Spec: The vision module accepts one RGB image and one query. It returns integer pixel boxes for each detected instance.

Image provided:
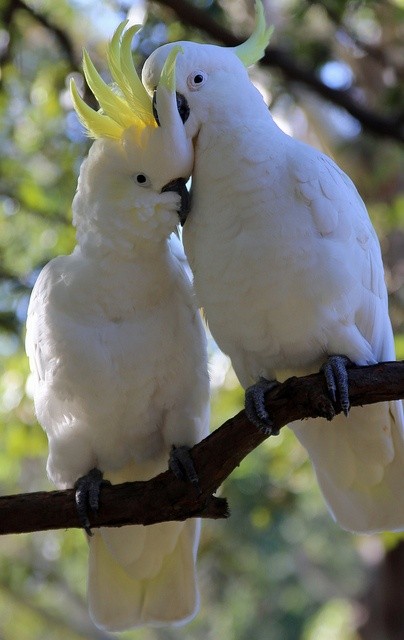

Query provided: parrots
[21,16,214,633]
[140,0,403,542]
